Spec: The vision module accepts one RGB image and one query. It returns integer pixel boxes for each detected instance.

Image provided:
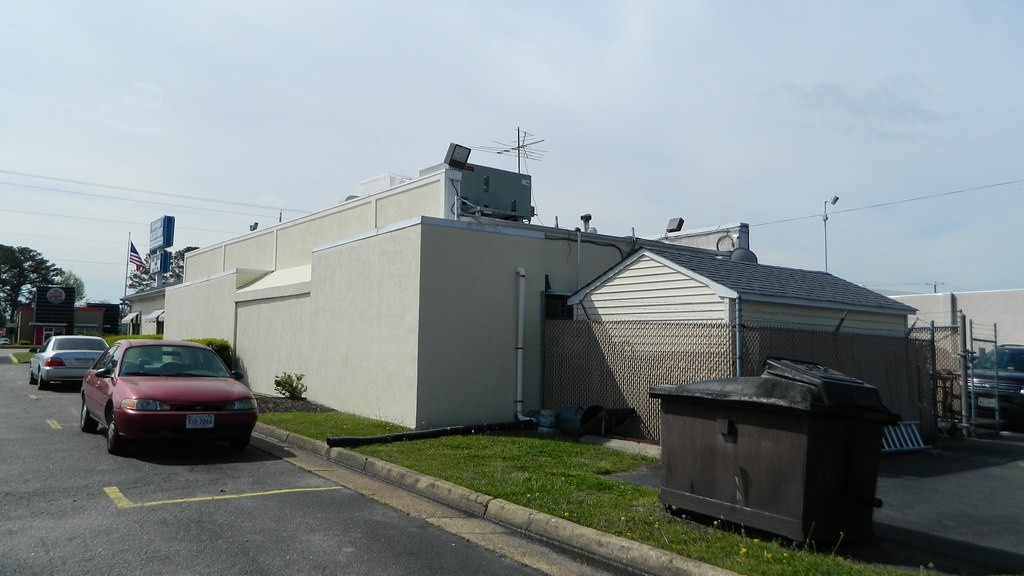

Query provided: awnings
[144,309,165,322]
[121,312,140,324]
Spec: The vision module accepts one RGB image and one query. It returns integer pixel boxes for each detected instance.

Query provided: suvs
[965,343,1024,424]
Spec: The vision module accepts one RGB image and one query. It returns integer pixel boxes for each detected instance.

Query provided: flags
[129,241,146,273]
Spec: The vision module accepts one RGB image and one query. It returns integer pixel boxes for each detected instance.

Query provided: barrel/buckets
[537,409,557,433]
[559,404,608,436]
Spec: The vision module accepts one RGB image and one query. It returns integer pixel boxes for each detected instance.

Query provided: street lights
[822,195,841,271]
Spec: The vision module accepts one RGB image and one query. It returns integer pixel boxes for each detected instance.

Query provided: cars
[78,339,259,457]
[0,338,11,345]
[28,335,112,390]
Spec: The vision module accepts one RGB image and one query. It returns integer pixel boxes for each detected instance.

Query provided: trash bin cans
[649,356,883,546]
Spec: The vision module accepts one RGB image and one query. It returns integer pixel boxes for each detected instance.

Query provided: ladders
[880,421,932,453]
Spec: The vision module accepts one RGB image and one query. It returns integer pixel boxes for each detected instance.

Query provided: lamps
[665,217,684,234]
[443,142,471,169]
